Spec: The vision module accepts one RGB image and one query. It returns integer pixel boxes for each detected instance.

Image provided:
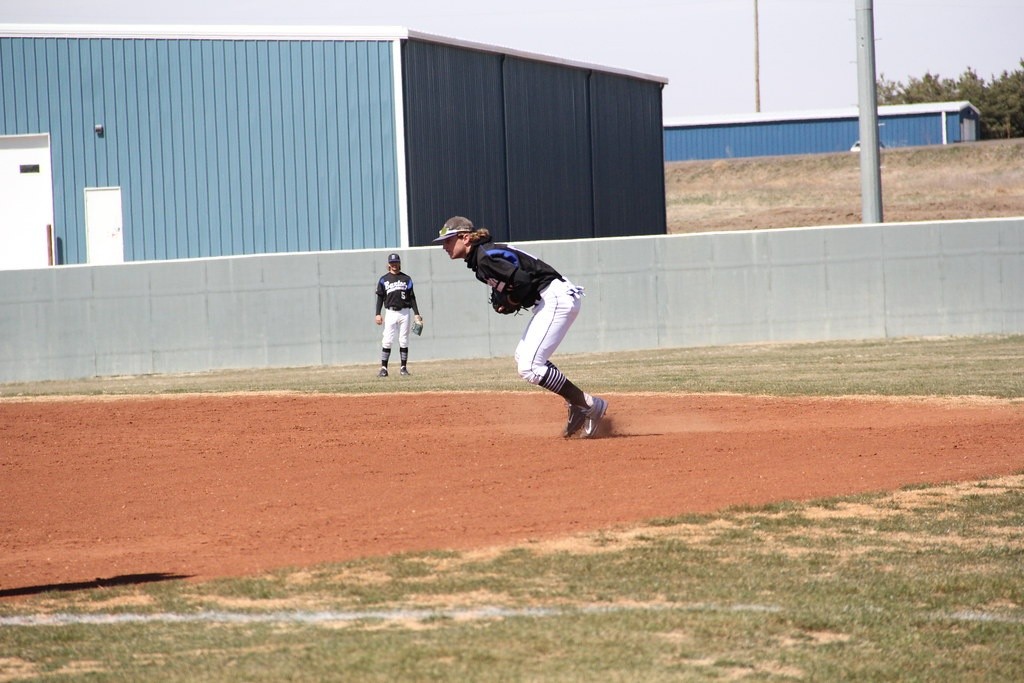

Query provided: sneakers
[562,400,586,437]
[577,396,608,438]
[377,368,388,377]
[400,368,410,375]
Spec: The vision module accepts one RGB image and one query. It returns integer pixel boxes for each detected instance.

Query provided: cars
[850,139,884,150]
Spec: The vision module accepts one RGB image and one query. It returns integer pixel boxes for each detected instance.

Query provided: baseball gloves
[411,315,424,337]
[489,286,520,316]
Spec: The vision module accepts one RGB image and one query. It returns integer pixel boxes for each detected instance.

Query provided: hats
[388,254,400,262]
[432,216,474,245]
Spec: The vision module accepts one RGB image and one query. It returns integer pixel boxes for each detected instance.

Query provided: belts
[388,306,402,311]
[534,277,566,306]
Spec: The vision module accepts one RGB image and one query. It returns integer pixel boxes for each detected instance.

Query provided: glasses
[440,229,470,236]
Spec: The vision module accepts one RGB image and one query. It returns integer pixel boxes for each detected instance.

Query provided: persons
[432,215,608,439]
[376,253,422,377]
